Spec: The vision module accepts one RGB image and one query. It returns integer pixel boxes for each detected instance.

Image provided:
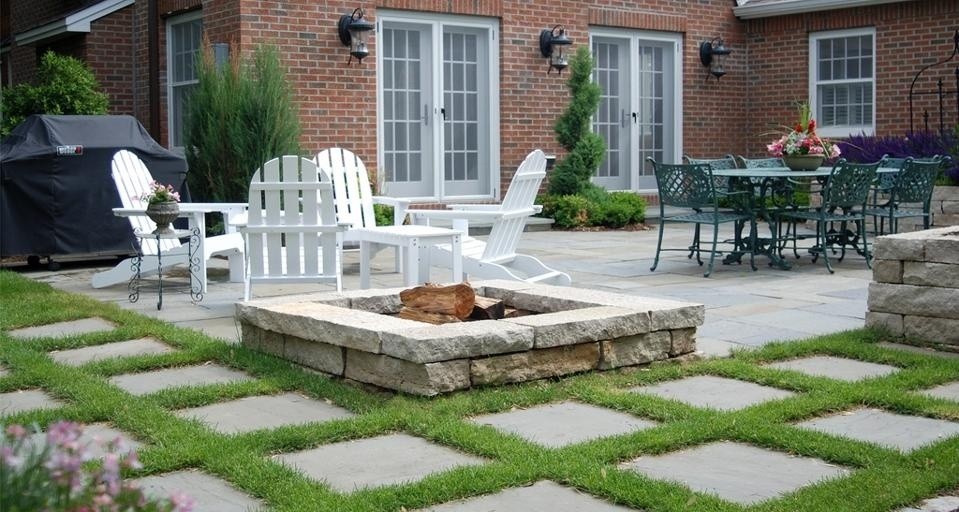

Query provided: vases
[781,155,825,171]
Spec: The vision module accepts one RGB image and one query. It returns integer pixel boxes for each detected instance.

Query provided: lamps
[699,36,733,83]
[537,23,574,78]
[336,5,376,68]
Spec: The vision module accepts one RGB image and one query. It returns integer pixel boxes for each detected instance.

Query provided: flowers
[753,89,844,164]
